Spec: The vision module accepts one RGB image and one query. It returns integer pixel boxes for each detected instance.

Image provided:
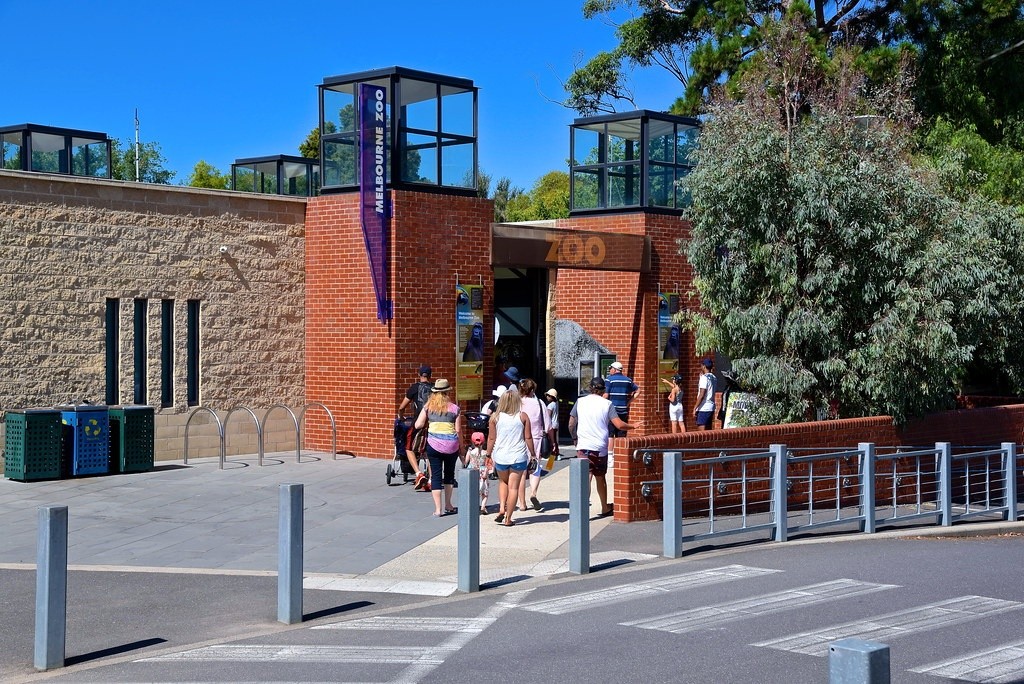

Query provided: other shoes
[433,507,458,518]
[607,455,614,462]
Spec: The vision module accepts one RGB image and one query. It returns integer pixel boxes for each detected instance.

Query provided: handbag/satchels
[541,436,550,458]
[411,428,428,452]
[528,456,537,474]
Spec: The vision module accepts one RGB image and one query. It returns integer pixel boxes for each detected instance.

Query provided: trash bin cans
[55,404,111,477]
[107,405,155,475]
[4,409,62,484]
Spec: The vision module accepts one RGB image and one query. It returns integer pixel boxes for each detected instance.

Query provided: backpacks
[418,381,433,409]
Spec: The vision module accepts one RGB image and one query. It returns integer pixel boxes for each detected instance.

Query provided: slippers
[602,503,614,516]
[494,497,541,526]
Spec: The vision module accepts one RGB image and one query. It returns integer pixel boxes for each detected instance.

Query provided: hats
[431,379,453,393]
[492,385,508,398]
[671,374,682,382]
[609,362,623,371]
[471,431,485,445]
[544,389,557,400]
[590,377,605,390]
[419,366,431,376]
[504,367,519,381]
[703,359,712,368]
[721,369,736,382]
[495,356,508,364]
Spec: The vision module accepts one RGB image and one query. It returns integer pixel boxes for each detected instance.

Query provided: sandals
[480,507,488,515]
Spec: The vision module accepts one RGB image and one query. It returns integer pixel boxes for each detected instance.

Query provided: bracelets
[460,445,465,449]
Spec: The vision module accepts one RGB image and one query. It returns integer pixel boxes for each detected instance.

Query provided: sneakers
[414,473,432,491]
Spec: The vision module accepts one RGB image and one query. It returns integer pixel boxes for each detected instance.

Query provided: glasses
[609,367,614,370]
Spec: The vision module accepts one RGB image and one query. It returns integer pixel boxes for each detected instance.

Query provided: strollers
[463,412,498,481]
[386,417,429,485]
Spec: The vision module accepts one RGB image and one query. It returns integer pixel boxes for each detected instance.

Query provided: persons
[414,379,466,517]
[464,363,559,527]
[661,374,686,434]
[716,369,743,429]
[602,362,641,468]
[693,358,718,431]
[398,366,434,490]
[567,377,644,517]
